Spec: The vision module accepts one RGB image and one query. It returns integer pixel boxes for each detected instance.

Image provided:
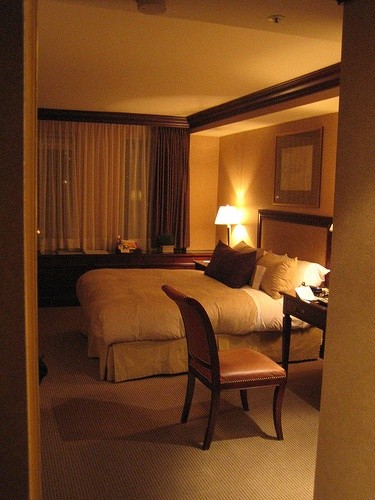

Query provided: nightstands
[278,284,330,374]
[192,258,212,269]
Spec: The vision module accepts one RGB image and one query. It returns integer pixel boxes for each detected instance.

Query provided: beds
[77,209,334,382]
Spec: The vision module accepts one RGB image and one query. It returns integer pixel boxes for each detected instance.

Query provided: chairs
[161,284,288,450]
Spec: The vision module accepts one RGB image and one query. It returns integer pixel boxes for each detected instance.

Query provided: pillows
[202,240,330,298]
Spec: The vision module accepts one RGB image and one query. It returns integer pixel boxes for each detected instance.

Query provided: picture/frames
[273,124,323,208]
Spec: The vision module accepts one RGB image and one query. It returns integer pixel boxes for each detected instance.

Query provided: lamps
[215,206,244,246]
